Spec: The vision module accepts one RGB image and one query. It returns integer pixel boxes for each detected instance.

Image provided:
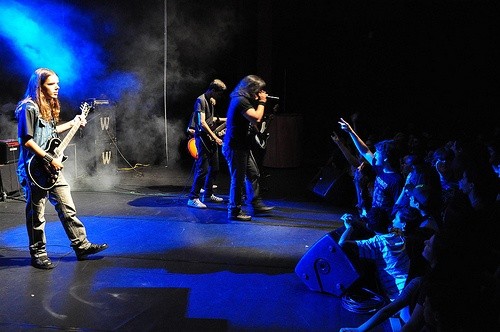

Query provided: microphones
[266,95,279,101]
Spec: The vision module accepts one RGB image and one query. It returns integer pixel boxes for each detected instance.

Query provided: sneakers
[207,194,223,202]
[187,198,207,208]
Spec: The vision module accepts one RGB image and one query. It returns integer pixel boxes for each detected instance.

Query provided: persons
[330,112,500,332]
[218,74,276,221]
[15,66,111,270]
[186,79,227,209]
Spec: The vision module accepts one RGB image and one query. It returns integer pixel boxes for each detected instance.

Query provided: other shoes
[251,206,274,214]
[32,256,55,268]
[227,213,251,220]
[73,243,108,261]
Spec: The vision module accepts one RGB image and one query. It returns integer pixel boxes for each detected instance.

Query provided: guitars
[188,128,226,159]
[255,103,279,149]
[28,102,90,190]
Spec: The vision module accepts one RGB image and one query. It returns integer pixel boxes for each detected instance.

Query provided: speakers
[83,104,117,175]
[295,220,378,296]
[0,162,21,195]
[312,172,357,209]
